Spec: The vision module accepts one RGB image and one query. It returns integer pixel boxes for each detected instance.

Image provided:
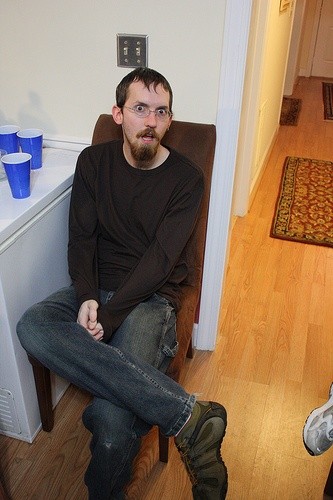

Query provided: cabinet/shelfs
[0,140,91,444]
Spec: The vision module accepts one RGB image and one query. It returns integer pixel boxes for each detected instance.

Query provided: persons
[18,66,227,500]
[302,380,333,500]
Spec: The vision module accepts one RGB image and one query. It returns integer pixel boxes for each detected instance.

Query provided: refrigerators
[0,134,92,446]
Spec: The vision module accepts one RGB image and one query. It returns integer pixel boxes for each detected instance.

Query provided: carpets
[280,96,302,126]
[322,81,333,120]
[270,156,333,248]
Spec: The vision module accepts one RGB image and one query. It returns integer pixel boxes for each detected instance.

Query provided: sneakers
[174,401,228,500]
[302,382,333,456]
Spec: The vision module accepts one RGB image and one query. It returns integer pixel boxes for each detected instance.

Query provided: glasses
[122,105,174,119]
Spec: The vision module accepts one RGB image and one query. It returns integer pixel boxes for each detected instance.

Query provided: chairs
[32,114,216,464]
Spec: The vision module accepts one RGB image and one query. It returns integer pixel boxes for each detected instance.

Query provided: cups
[0,125,21,154]
[1,152,33,199]
[0,149,8,182]
[16,129,43,170]
